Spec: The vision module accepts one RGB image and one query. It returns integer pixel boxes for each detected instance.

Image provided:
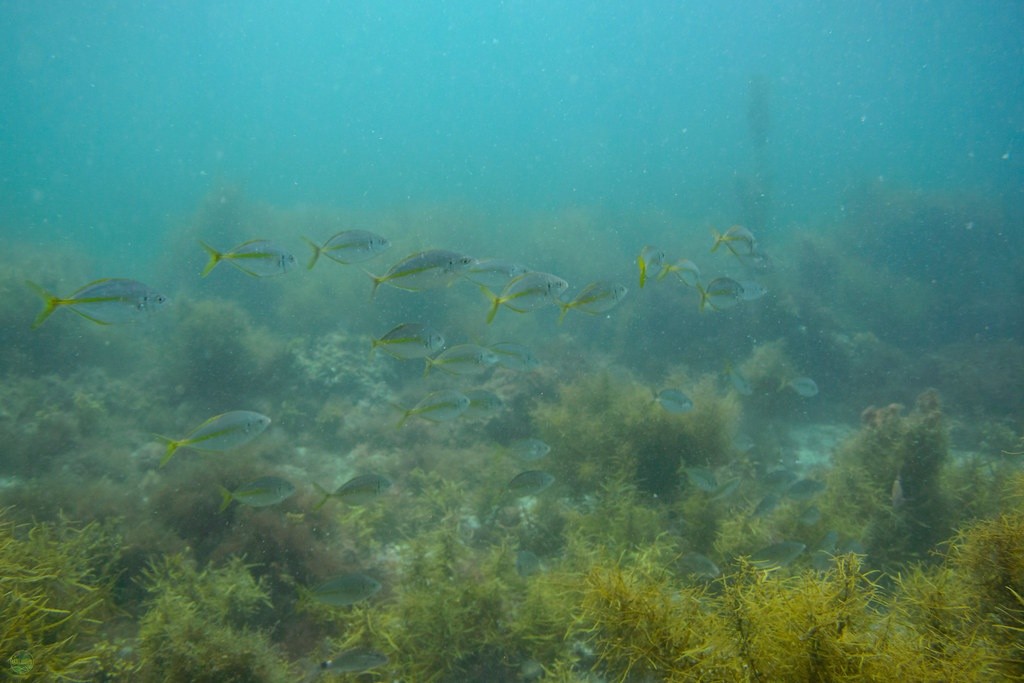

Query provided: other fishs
[297,223,828,683]
[312,475,392,515]
[392,389,471,432]
[198,240,300,282]
[369,322,445,360]
[304,229,392,272]
[153,410,271,468]
[214,475,298,516]
[24,278,166,330]
[365,248,479,297]
